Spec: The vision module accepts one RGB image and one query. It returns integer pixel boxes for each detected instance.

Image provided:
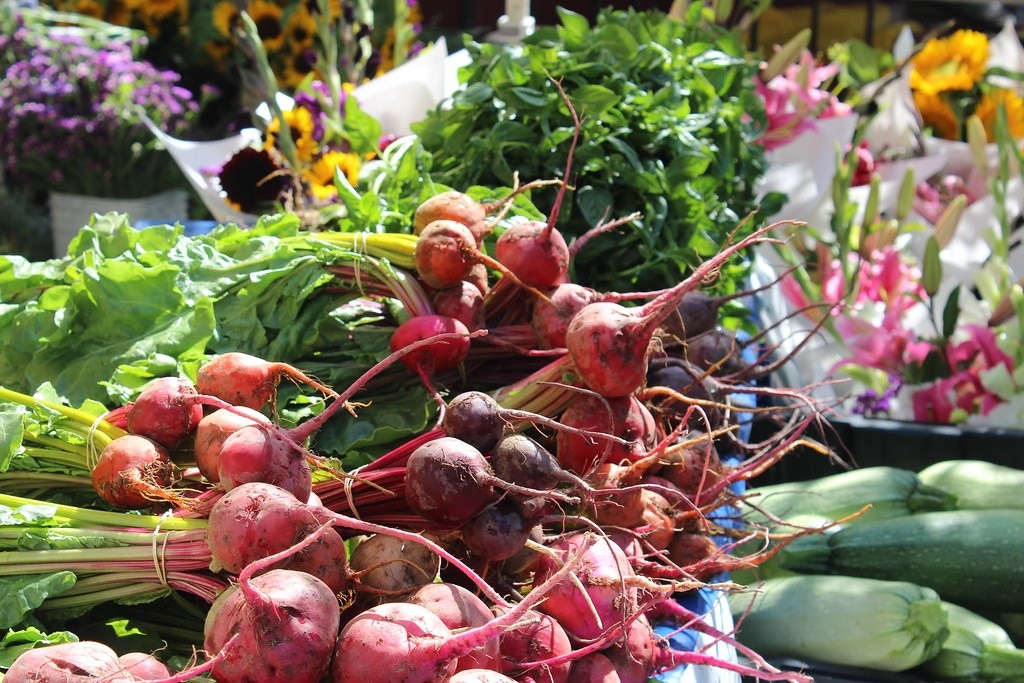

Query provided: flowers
[0,0,425,217]
[752,21,1023,426]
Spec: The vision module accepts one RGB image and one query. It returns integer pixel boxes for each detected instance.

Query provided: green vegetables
[394,1,792,305]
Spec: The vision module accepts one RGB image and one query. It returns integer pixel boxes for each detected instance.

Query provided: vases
[50,190,189,260]
